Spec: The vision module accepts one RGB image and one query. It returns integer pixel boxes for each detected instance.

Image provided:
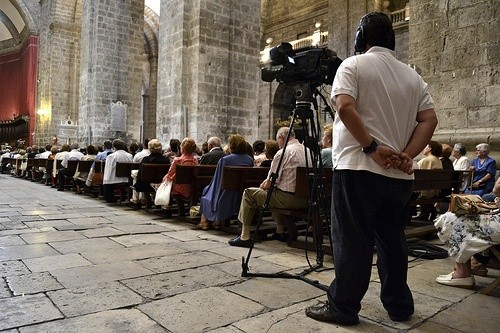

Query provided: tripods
[241,104,334,276]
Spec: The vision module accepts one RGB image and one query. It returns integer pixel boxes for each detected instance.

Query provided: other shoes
[196,223,208,230]
[229,236,254,247]
[271,233,288,242]
[435,271,476,289]
[470,264,488,277]
[50,185,56,188]
[130,198,140,203]
[413,213,429,220]
[210,223,220,230]
[75,191,82,194]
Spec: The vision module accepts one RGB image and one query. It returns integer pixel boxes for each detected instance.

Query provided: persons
[305,13,439,324]
[0,122,500,290]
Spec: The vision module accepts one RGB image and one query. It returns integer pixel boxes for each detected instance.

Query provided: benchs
[1,157,465,255]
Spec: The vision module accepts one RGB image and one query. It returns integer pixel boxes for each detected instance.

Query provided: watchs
[361,139,379,156]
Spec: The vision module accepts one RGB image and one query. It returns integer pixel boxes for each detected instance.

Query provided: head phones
[355,13,396,52]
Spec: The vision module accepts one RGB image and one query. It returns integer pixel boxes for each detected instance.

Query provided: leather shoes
[305,299,360,326]
[387,311,411,321]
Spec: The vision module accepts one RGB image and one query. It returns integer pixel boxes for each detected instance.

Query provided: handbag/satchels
[448,194,498,215]
[190,205,200,215]
[155,182,172,205]
